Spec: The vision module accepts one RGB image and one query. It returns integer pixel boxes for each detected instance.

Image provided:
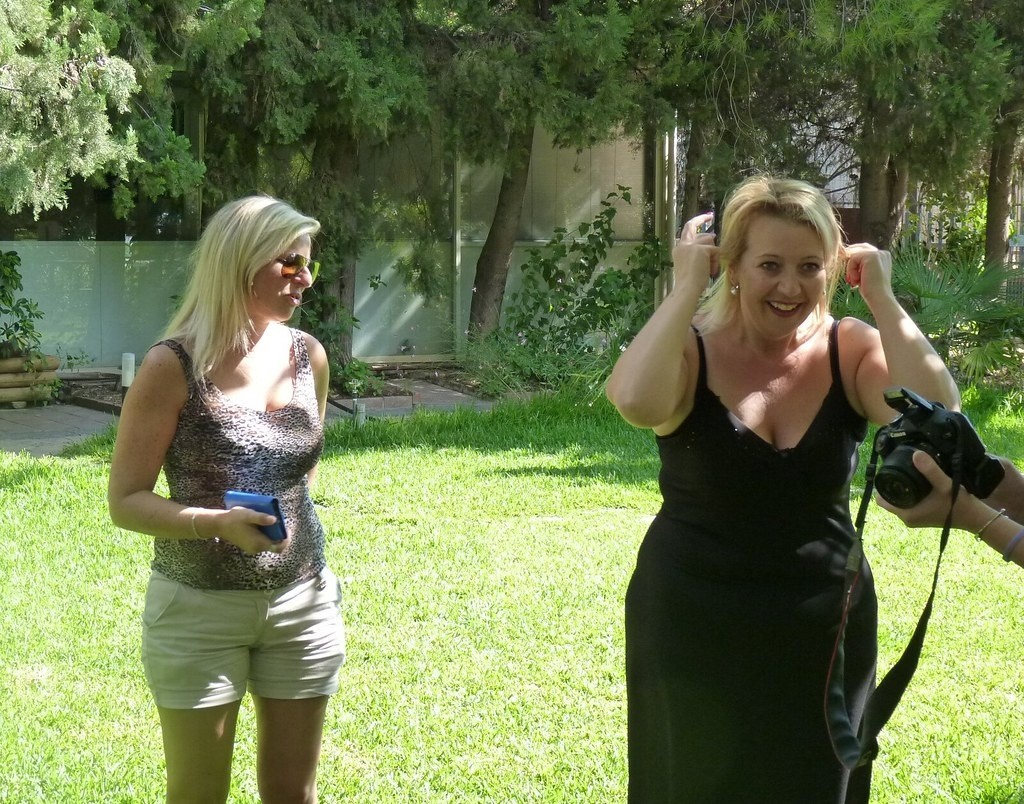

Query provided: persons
[106,197,345,804]
[605,174,1024,804]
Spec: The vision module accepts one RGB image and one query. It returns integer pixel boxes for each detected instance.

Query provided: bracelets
[1002,527,1024,562]
[191,507,209,541]
[969,508,1006,542]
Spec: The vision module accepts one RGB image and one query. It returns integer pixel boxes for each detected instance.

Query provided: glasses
[275,251,320,286]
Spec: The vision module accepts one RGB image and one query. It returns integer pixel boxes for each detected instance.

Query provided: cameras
[873,387,1005,509]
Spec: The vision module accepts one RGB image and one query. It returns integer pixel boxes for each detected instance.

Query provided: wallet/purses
[223,490,288,542]
